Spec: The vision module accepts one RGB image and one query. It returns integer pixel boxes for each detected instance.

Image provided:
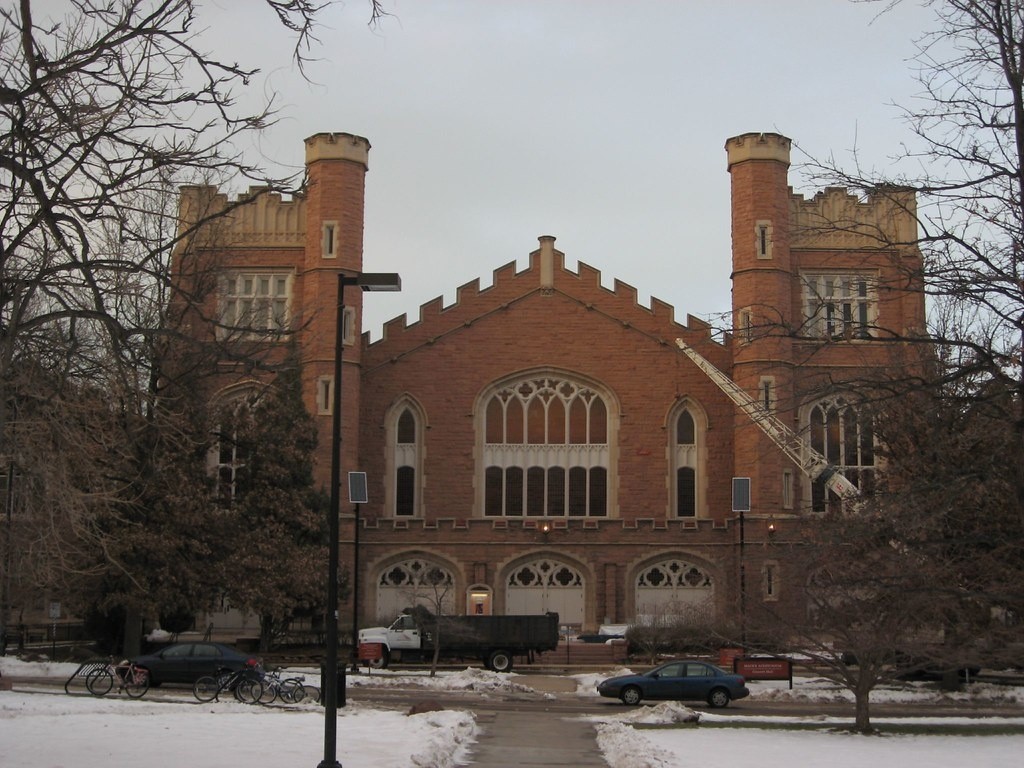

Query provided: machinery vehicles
[675,338,1015,676]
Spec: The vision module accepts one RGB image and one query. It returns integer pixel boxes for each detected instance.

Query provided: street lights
[315,272,401,768]
[348,471,368,671]
[732,477,750,682]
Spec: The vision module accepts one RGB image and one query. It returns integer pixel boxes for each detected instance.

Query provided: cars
[596,660,750,708]
[115,641,265,688]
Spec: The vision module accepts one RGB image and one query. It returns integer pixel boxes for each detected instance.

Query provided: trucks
[359,604,559,673]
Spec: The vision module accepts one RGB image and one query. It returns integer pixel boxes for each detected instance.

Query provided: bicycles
[86,655,149,699]
[193,663,321,707]
